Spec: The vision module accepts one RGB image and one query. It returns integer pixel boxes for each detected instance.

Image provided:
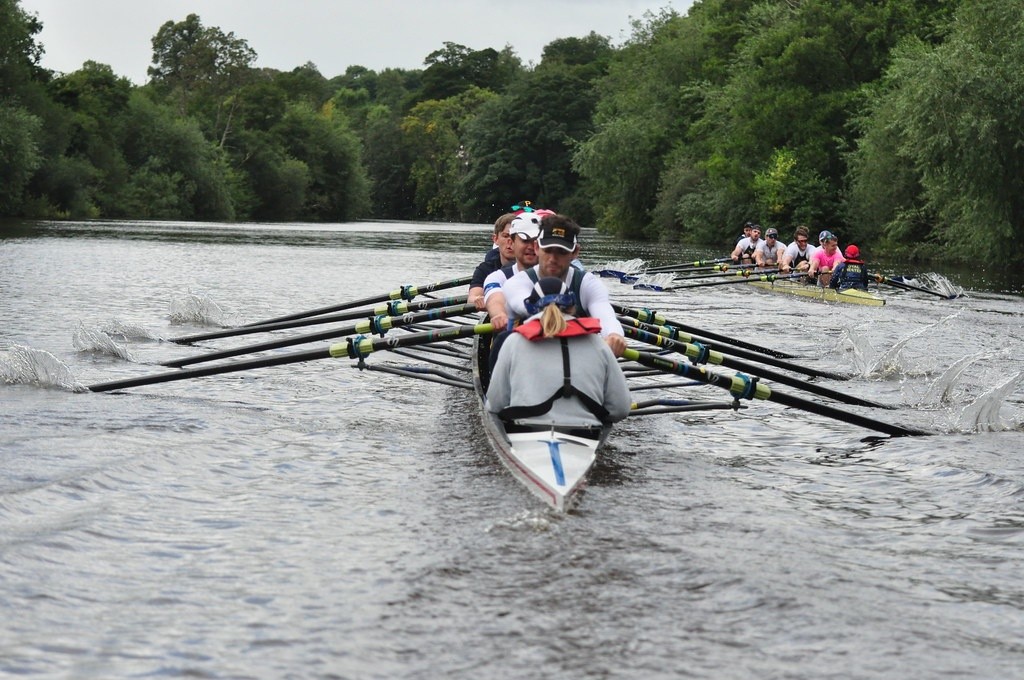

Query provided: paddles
[85,319,518,394]
[620,345,934,437]
[166,275,476,344]
[591,257,835,293]
[632,270,833,294]
[610,301,901,410]
[157,302,489,368]
[867,271,968,300]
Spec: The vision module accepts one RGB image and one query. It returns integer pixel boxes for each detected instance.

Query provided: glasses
[511,206,534,213]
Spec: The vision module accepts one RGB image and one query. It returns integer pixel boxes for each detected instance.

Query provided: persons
[829,245,867,292]
[731,222,764,270]
[755,227,787,270]
[779,227,816,274]
[486,278,631,441]
[467,208,627,393]
[808,231,847,286]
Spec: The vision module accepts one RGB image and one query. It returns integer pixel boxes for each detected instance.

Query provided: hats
[844,245,860,258]
[766,228,778,238]
[818,231,832,241]
[509,201,538,215]
[751,225,763,231]
[744,222,753,228]
[533,209,556,218]
[537,214,581,253]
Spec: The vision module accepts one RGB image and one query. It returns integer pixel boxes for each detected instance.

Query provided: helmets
[524,277,578,315]
[509,213,544,241]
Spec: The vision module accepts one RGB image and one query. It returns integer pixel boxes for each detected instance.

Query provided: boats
[472,310,614,520]
[714,260,889,309]
